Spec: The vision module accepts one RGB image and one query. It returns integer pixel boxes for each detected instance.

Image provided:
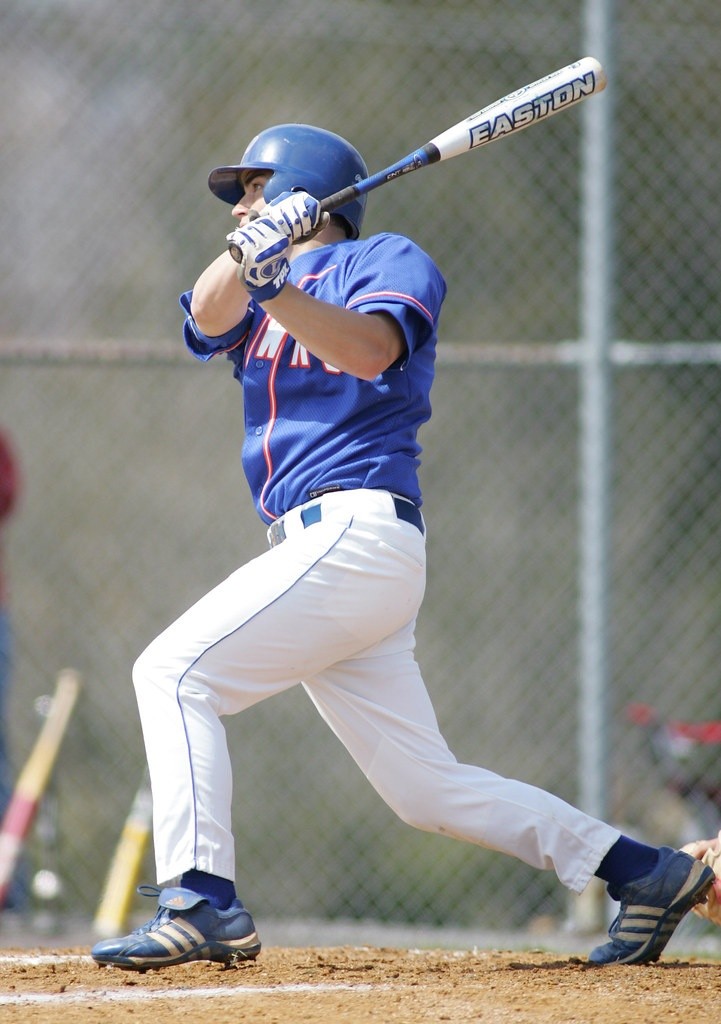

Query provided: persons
[91,125,715,968]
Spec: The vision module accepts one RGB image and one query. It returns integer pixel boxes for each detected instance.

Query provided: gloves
[225,216,294,303]
[258,191,330,246]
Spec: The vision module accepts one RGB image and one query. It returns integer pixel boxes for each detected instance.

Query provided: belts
[269,496,424,549]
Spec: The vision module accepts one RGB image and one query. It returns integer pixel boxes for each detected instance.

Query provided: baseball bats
[226,52,610,264]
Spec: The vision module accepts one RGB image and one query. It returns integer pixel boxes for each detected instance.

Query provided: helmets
[208,123,370,240]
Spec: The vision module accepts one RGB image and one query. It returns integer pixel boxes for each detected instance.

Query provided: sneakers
[588,845,715,965]
[92,885,262,972]
